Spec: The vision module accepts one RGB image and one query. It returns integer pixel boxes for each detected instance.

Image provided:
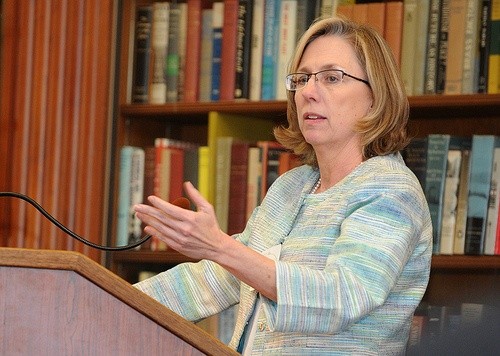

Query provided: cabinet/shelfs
[101,0,500,356]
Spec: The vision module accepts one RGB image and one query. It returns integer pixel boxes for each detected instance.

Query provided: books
[116,0,499,106]
[134,269,238,345]
[115,109,500,256]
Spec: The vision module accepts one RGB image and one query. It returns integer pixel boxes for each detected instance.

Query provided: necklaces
[310,176,323,197]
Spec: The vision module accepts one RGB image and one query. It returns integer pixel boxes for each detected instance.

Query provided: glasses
[286,69,370,91]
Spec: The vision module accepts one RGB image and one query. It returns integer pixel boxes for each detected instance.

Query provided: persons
[125,16,432,356]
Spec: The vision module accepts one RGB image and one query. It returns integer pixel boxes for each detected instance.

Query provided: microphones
[0,192,192,251]
